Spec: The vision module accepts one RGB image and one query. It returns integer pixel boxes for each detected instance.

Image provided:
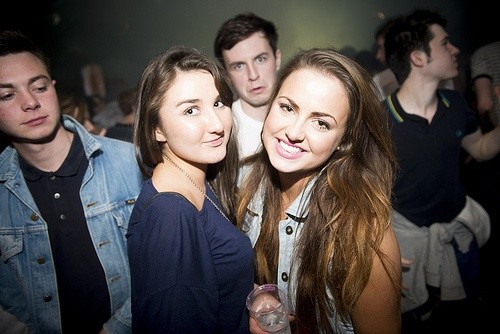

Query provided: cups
[245,284,291,334]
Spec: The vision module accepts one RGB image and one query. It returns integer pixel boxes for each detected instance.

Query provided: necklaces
[161,152,230,222]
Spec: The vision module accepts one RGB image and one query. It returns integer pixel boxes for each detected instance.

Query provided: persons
[0,31,145,334]
[214,12,281,191]
[124,46,255,334]
[372,8,500,334]
[60,88,139,144]
[235,48,402,334]
